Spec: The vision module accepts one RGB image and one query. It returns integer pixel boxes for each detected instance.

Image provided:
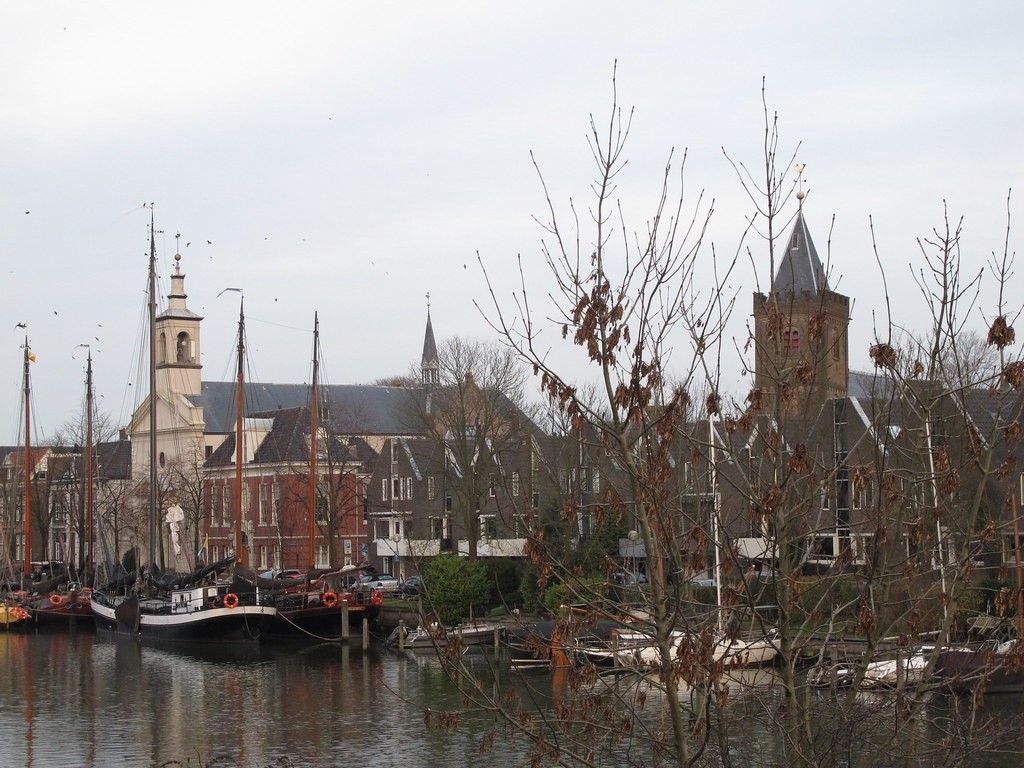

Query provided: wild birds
[15,201,280,386]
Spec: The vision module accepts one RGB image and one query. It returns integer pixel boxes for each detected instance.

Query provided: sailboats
[0,202,384,644]
[808,415,973,688]
[574,413,782,674]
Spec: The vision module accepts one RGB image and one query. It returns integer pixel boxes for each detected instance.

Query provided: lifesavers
[322,591,338,608]
[372,591,384,606]
[223,593,239,608]
[50,594,63,605]
[10,606,29,619]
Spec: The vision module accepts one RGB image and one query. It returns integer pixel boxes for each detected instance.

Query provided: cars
[282,572,318,595]
[398,575,423,594]
[689,579,723,589]
[351,572,399,600]
[608,569,647,586]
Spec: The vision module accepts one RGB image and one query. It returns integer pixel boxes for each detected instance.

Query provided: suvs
[259,569,301,579]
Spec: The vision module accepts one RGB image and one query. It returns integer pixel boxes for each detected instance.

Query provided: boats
[494,618,619,662]
[384,621,508,649]
[560,600,652,624]
